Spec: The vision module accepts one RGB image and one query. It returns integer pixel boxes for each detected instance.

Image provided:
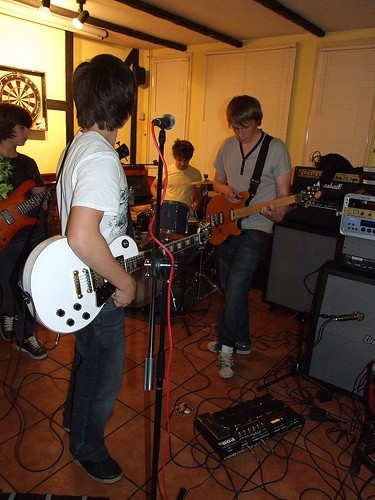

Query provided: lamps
[74,0,90,30]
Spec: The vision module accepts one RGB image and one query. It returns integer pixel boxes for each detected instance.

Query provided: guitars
[0,140,129,253]
[23,222,214,334]
[205,186,324,245]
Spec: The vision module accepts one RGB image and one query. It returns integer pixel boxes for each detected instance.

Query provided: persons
[54,52,137,484]
[0,102,49,359]
[207,94,292,378]
[150,139,203,213]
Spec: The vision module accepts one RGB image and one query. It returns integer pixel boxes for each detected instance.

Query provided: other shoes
[70,454,120,483]
[62,424,70,432]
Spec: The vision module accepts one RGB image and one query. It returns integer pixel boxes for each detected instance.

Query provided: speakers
[261,202,375,400]
[125,173,153,204]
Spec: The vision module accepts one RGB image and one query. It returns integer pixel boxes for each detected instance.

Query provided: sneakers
[0,314,19,340]
[207,342,251,355]
[16,335,48,359]
[217,349,234,378]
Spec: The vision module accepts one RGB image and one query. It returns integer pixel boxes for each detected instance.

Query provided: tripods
[183,245,224,304]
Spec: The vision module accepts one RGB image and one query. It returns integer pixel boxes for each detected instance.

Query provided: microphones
[329,311,364,322]
[152,114,175,130]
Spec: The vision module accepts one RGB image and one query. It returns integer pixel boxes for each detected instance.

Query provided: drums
[151,200,190,239]
[123,240,163,310]
